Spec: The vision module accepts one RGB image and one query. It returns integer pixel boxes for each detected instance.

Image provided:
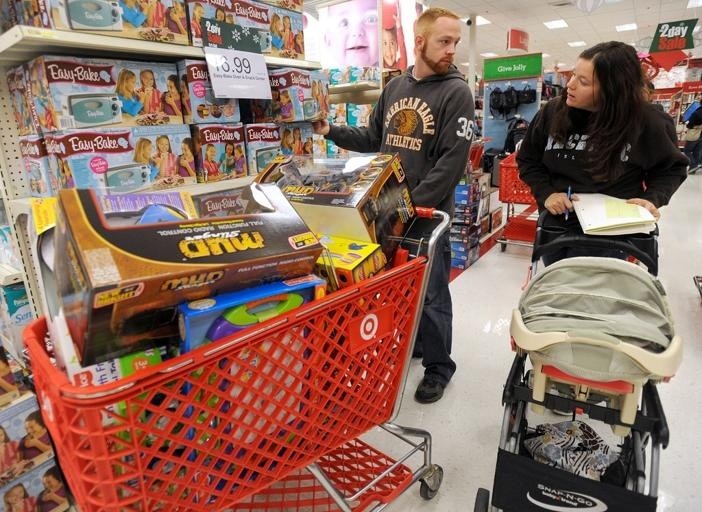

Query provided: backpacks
[504,117,530,152]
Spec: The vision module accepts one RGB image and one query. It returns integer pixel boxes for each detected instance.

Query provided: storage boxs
[450,168,504,268]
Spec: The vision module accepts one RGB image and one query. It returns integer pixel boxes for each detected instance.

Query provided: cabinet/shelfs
[0,25,323,368]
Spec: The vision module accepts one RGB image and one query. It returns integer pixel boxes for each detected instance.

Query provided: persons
[133,135,193,183]
[381,0,408,70]
[313,7,474,405]
[642,79,664,112]
[0,346,71,512]
[269,14,305,53]
[272,88,295,123]
[190,4,236,38]
[683,99,702,175]
[204,143,246,180]
[322,0,379,66]
[514,40,692,268]
[179,73,192,115]
[115,69,182,117]
[281,127,312,154]
[122,3,187,34]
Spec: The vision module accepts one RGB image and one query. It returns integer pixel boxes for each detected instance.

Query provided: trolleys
[21,206,455,512]
[467,135,494,172]
[491,146,546,258]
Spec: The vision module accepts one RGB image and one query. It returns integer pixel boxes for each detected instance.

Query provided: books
[570,191,658,238]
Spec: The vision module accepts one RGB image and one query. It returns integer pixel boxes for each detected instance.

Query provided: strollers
[469,194,689,512]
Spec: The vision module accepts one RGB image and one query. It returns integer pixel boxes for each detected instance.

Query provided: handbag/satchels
[489,85,536,120]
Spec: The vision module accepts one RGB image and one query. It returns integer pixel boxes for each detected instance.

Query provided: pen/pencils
[565,185,571,220]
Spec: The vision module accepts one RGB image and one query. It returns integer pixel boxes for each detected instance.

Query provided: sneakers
[687,164,702,174]
[416,369,447,403]
